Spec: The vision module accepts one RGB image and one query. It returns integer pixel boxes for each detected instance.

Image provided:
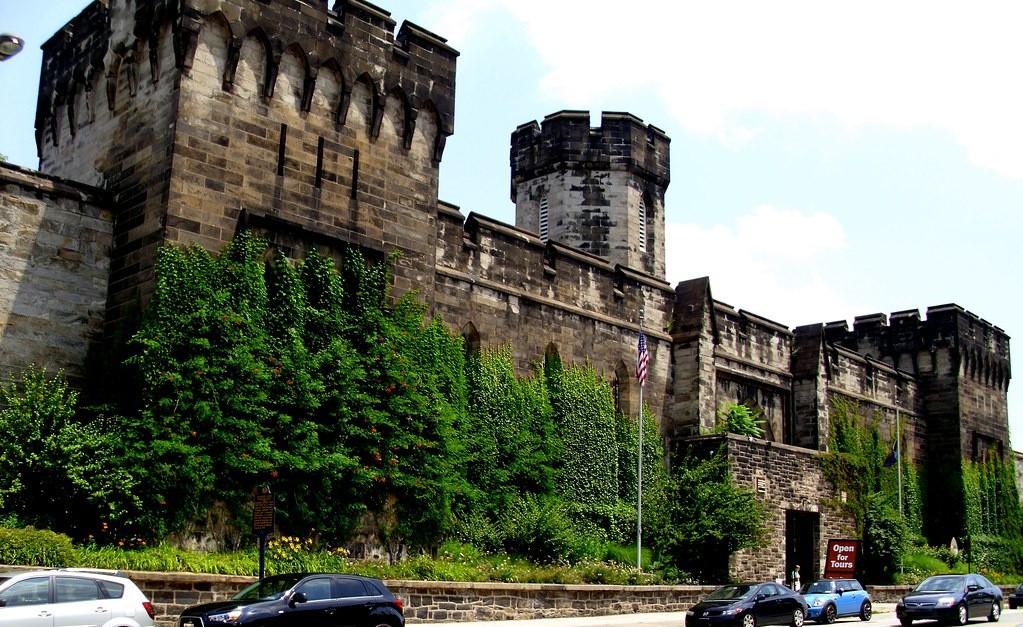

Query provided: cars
[178,573,405,627]
[896,575,1004,626]
[1009,582,1023,609]
[797,579,872,623]
[685,582,808,627]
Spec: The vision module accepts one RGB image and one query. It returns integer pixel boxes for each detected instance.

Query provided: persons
[791,565,800,592]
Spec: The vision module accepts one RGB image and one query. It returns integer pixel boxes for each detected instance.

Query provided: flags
[635,327,650,388]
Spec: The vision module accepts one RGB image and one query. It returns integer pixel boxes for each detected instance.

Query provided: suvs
[0,568,155,627]
